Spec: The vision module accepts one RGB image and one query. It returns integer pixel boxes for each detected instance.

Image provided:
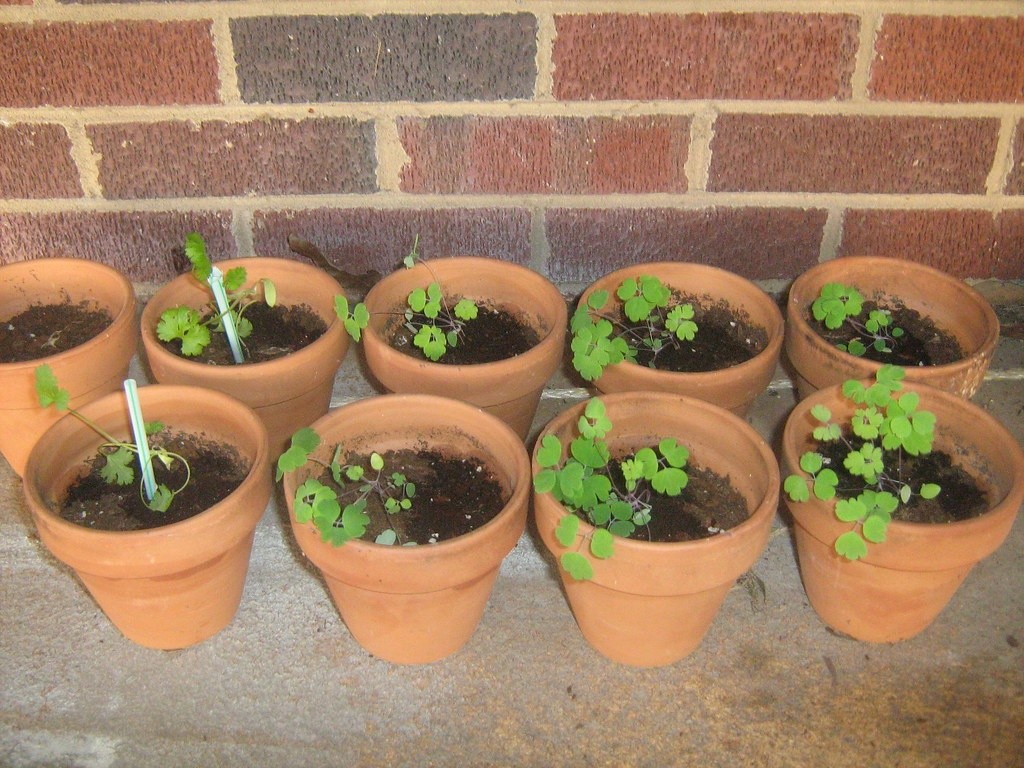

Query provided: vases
[0,256,140,479]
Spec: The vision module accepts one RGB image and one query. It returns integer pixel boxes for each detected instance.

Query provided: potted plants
[276,392,532,666]
[333,230,567,446]
[139,231,350,464]
[780,364,1023,647]
[22,363,273,650]
[784,252,1001,403]
[532,391,781,667]
[568,261,786,424]
